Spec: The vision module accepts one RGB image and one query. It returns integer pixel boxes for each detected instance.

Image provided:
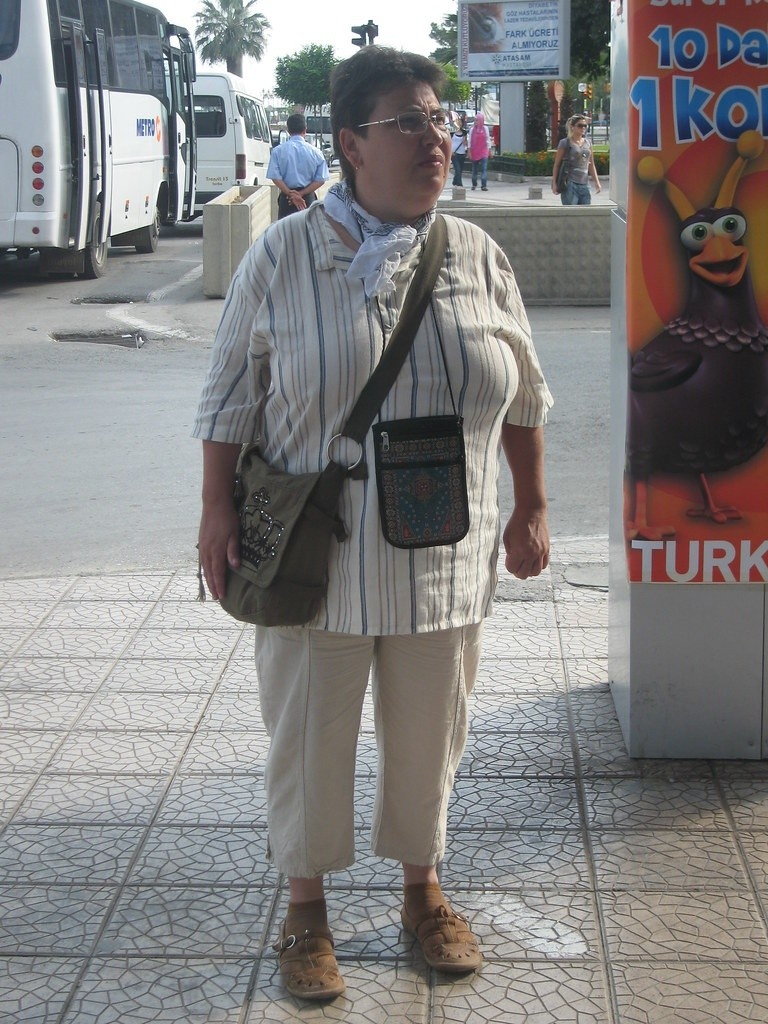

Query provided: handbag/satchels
[208,444,346,628]
[371,412,471,549]
[550,137,570,194]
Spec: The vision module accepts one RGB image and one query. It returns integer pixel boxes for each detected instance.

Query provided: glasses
[346,110,465,136]
[573,123,588,128]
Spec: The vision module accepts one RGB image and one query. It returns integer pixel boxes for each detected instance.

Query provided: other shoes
[482,186,487,190]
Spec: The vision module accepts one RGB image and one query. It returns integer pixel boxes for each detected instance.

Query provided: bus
[0,0,197,278]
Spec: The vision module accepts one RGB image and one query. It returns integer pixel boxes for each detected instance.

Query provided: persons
[551,114,602,205]
[450,114,492,191]
[191,47,552,997]
[266,113,328,219]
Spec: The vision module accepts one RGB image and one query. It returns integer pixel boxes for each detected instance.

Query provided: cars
[591,120,609,126]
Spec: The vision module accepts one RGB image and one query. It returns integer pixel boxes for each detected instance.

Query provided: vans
[455,107,482,134]
[166,70,287,204]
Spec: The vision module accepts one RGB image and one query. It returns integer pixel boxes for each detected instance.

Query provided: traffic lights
[365,23,379,37]
[586,84,593,99]
[351,25,366,47]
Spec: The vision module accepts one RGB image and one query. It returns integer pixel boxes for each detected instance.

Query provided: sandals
[273,917,344,1000]
[399,903,482,972]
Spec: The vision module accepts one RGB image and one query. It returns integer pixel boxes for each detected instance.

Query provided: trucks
[302,101,335,159]
